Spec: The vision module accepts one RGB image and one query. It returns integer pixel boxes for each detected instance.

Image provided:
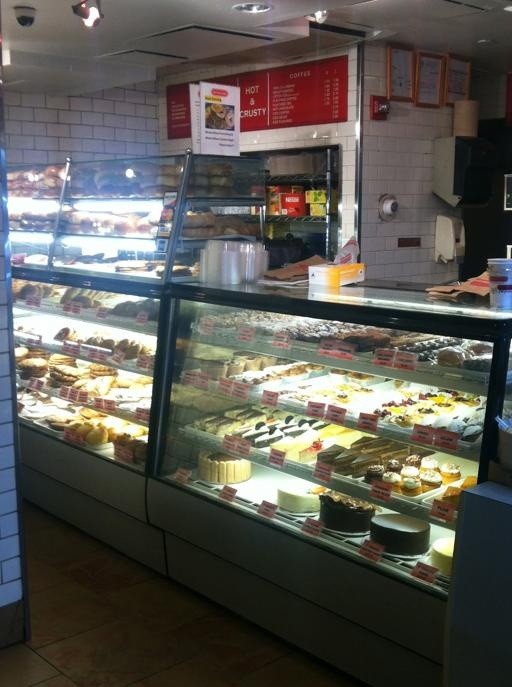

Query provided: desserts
[180,351,486,450]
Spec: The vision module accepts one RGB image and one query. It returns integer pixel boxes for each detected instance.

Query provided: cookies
[47,364,80,389]
[18,358,48,380]
[88,362,118,382]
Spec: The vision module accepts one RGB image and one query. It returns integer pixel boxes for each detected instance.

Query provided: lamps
[1,44,21,74]
[77,4,104,33]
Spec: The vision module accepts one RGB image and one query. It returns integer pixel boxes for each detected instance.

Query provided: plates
[356,471,466,503]
[307,459,365,483]
[64,432,149,449]
[420,481,466,517]
[32,410,82,437]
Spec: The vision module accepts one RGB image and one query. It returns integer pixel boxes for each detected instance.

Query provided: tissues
[308,262,365,287]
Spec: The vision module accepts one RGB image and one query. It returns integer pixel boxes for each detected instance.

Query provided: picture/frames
[415,48,446,108]
[387,41,419,102]
[442,53,474,106]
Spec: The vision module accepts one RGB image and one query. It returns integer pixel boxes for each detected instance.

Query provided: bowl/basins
[199,239,270,285]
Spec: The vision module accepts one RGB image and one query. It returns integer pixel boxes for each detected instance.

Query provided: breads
[20,284,51,300]
[61,287,108,309]
[65,420,120,445]
[54,327,74,343]
[83,334,139,359]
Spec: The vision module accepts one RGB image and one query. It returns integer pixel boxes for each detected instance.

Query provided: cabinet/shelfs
[7,149,510,684]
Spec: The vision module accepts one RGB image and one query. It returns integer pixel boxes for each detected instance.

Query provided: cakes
[422,456,439,472]
[313,440,383,484]
[387,458,399,469]
[370,514,430,557]
[277,483,328,514]
[442,486,462,511]
[441,464,458,483]
[320,490,380,533]
[431,534,455,577]
[401,466,422,478]
[406,454,422,468]
[383,472,400,486]
[421,472,440,488]
[402,479,422,496]
[197,447,252,484]
[461,476,477,491]
